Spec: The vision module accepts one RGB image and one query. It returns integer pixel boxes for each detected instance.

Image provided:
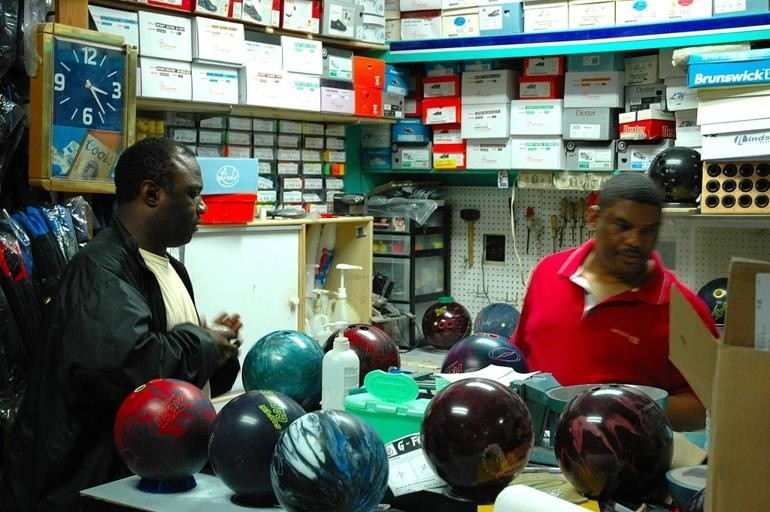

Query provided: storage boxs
[509,100,564,136]
[433,145,466,170]
[382,93,405,118]
[243,31,283,75]
[399,2,441,11]
[625,56,665,84]
[625,83,668,112]
[355,87,385,119]
[138,58,192,103]
[404,92,423,118]
[509,136,567,170]
[713,0,768,18]
[138,11,192,62]
[283,0,321,34]
[422,98,461,126]
[441,1,478,8]
[465,139,509,169]
[391,119,431,144]
[142,0,195,13]
[463,58,496,71]
[323,48,354,82]
[320,79,355,117]
[384,64,407,95]
[238,66,284,109]
[670,0,712,19]
[523,2,568,34]
[361,125,391,148]
[384,14,399,40]
[423,77,460,97]
[194,0,242,20]
[565,139,616,171]
[344,370,429,442]
[696,87,769,126]
[352,56,384,89]
[665,78,698,111]
[88,5,140,57]
[674,111,700,146]
[391,143,433,169]
[524,56,567,75]
[370,207,452,347]
[243,0,280,29]
[432,125,463,144]
[408,73,423,96]
[321,0,363,39]
[569,0,615,29]
[285,73,321,112]
[519,77,564,99]
[461,104,511,139]
[668,257,770,512]
[659,49,688,77]
[616,0,670,26]
[201,193,257,224]
[386,1,398,10]
[619,138,675,173]
[197,158,256,195]
[564,72,626,108]
[362,148,392,170]
[479,2,523,36]
[461,70,521,104]
[281,37,324,76]
[563,109,619,140]
[191,17,242,68]
[191,65,238,106]
[619,109,674,140]
[442,8,479,39]
[567,53,624,72]
[700,118,770,161]
[688,48,769,91]
[400,12,441,42]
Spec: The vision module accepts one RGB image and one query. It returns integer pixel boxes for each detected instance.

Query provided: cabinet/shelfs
[158,218,375,389]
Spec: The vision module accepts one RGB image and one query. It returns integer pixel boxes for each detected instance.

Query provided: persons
[510,172,720,433]
[0,138,244,511]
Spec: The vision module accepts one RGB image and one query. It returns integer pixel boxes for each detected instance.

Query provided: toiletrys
[307,289,329,342]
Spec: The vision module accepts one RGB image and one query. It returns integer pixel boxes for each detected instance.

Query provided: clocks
[29,25,138,195]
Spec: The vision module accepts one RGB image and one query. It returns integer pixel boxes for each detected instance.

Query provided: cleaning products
[304,288,333,347]
